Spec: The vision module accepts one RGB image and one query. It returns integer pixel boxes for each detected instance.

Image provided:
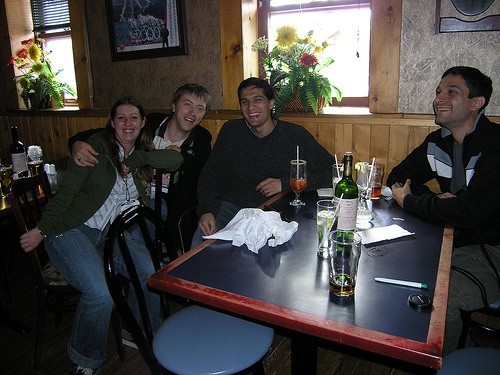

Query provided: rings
[78,158,81,162]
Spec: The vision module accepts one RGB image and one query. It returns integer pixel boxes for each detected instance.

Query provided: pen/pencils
[373,277,427,288]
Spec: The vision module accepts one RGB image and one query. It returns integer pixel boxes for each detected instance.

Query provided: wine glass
[290,160,307,207]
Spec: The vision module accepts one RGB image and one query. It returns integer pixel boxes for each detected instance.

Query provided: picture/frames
[106,0,189,62]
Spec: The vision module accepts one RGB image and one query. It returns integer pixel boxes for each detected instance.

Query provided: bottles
[9,126,28,178]
[354,162,373,223]
[44,164,55,175]
[334,153,358,253]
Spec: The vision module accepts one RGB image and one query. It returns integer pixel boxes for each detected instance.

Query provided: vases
[276,85,326,113]
[27,91,63,109]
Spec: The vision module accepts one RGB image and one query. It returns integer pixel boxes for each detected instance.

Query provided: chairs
[0,137,500,375]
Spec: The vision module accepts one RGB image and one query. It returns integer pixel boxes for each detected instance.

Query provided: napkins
[355,224,416,245]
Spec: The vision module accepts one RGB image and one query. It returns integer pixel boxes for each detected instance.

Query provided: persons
[190,77,336,250]
[387,67,500,324]
[21,84,212,375]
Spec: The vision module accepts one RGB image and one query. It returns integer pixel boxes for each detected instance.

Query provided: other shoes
[72,365,97,375]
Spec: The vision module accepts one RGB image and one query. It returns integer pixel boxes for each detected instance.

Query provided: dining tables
[148,184,454,375]
[0,170,57,221]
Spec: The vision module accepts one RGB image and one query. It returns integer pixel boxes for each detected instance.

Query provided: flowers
[251,25,342,116]
[6,37,79,109]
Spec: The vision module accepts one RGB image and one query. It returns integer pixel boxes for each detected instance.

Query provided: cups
[333,164,342,192]
[0,164,13,196]
[328,230,361,296]
[316,200,340,257]
[368,162,384,199]
